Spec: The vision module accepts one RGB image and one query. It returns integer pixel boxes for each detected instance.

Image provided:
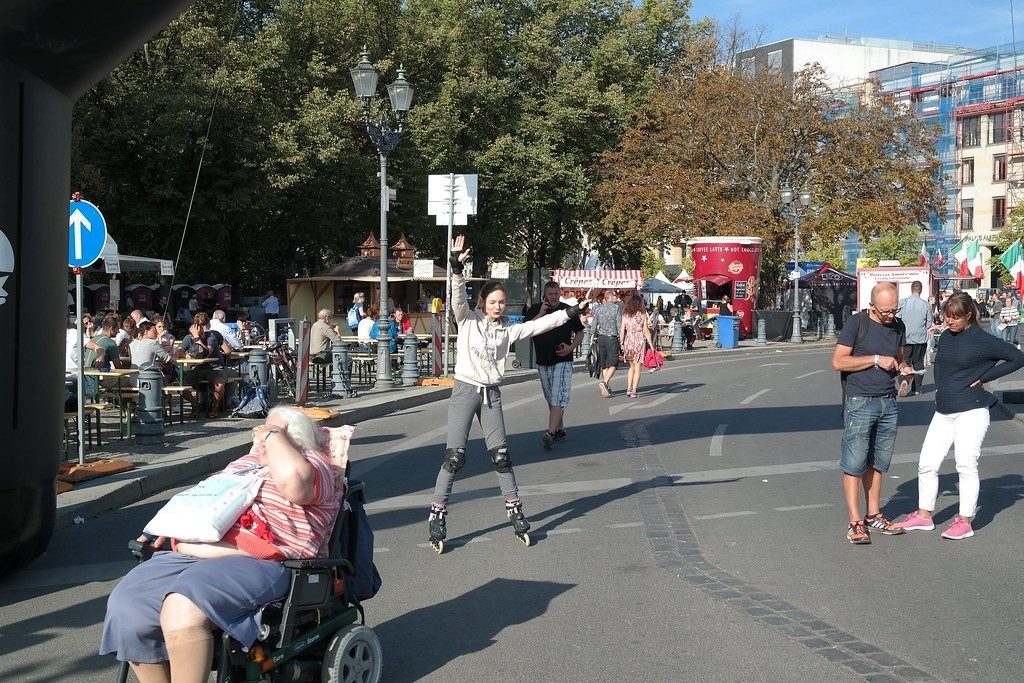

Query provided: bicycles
[250,322,298,397]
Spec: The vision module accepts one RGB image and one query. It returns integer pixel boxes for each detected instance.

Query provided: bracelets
[874,355,879,368]
[265,429,279,440]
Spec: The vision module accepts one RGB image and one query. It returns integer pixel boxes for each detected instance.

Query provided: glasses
[871,301,901,316]
[84,319,91,326]
[326,315,331,319]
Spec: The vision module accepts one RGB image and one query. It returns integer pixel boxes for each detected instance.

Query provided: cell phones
[543,295,548,303]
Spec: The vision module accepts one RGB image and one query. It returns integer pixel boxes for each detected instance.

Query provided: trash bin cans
[698,315,740,348]
[511,338,536,369]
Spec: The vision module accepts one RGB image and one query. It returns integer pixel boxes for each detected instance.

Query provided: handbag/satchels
[345,480,381,601]
[140,464,265,543]
[347,301,358,328]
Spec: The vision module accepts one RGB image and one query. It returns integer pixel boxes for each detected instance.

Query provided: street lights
[349,43,415,393]
[778,177,814,345]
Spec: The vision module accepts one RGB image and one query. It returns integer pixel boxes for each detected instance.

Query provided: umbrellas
[641,277,682,303]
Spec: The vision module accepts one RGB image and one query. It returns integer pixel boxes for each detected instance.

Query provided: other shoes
[189,402,202,417]
[126,414,140,423]
[924,362,933,367]
[912,390,923,395]
[171,404,186,413]
[600,380,612,399]
[1017,344,1021,351]
[627,389,639,398]
[208,402,219,419]
[899,380,908,397]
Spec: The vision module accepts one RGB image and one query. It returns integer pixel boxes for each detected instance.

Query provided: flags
[999,240,1024,295]
[920,239,927,266]
[936,243,944,264]
[951,235,985,287]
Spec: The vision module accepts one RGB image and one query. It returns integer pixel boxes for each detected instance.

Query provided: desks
[64,333,458,446]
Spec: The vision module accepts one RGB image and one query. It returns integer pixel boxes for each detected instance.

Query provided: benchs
[656,324,688,351]
[64,349,458,460]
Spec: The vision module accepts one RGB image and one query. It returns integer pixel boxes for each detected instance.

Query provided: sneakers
[554,427,567,443]
[863,514,904,535]
[941,517,975,539]
[543,431,554,450]
[847,520,870,544]
[894,511,936,530]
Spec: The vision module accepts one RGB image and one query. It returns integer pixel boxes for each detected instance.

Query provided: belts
[599,334,617,337]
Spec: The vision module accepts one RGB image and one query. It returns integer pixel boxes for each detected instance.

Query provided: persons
[894,292,1024,539]
[590,290,733,397]
[65,293,252,418]
[310,309,352,377]
[352,289,435,368]
[429,235,595,554]
[895,281,948,397]
[99,407,334,683]
[523,281,585,450]
[977,288,1021,350]
[262,291,279,319]
[834,282,915,543]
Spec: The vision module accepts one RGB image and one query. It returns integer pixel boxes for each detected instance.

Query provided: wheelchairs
[114,461,382,683]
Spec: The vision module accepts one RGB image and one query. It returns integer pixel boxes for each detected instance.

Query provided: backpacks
[230,385,270,418]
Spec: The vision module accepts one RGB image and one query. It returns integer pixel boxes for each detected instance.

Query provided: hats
[187,342,209,359]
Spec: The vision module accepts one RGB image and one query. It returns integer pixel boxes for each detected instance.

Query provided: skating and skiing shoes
[428,502,447,554]
[504,499,531,547]
